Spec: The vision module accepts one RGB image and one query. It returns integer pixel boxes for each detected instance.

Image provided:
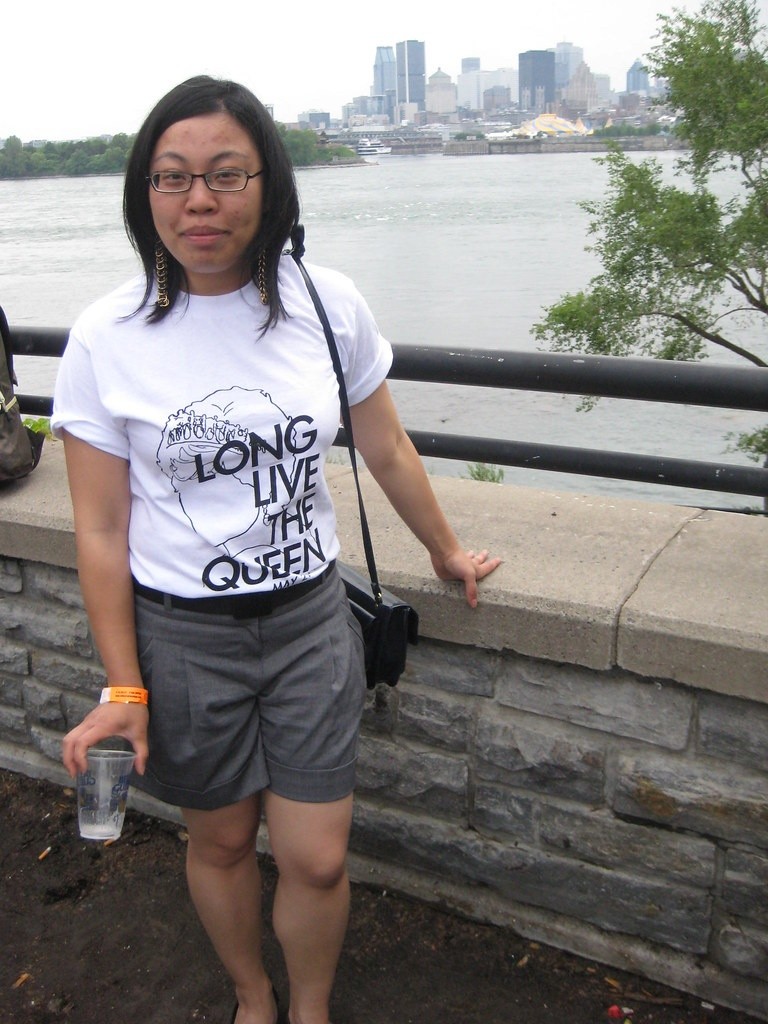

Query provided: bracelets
[96,685,151,706]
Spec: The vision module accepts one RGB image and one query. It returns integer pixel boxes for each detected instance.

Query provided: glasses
[146,166,264,192]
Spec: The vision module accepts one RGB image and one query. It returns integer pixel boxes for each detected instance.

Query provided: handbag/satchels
[334,557,420,691]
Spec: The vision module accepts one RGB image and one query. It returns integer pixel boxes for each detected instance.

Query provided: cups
[76,750,138,840]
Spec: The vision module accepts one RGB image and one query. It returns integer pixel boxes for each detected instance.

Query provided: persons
[44,67,501,1023]
